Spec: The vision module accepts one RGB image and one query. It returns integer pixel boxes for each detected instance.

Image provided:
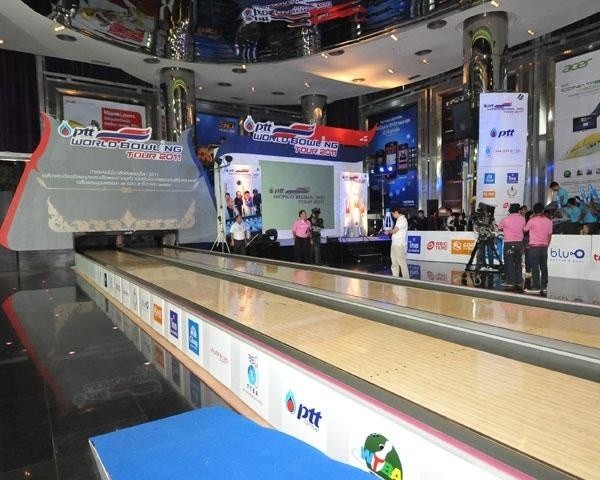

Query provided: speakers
[427,199,438,218]
[451,99,477,140]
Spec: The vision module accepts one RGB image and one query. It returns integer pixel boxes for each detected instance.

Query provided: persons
[417,178,600,235]
[229,214,251,255]
[498,203,527,287]
[291,210,311,263]
[522,210,534,276]
[305,206,324,264]
[225,188,261,218]
[522,203,554,293]
[382,205,410,280]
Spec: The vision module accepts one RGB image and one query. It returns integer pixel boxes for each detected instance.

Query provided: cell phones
[369,141,417,181]
[572,115,597,132]
